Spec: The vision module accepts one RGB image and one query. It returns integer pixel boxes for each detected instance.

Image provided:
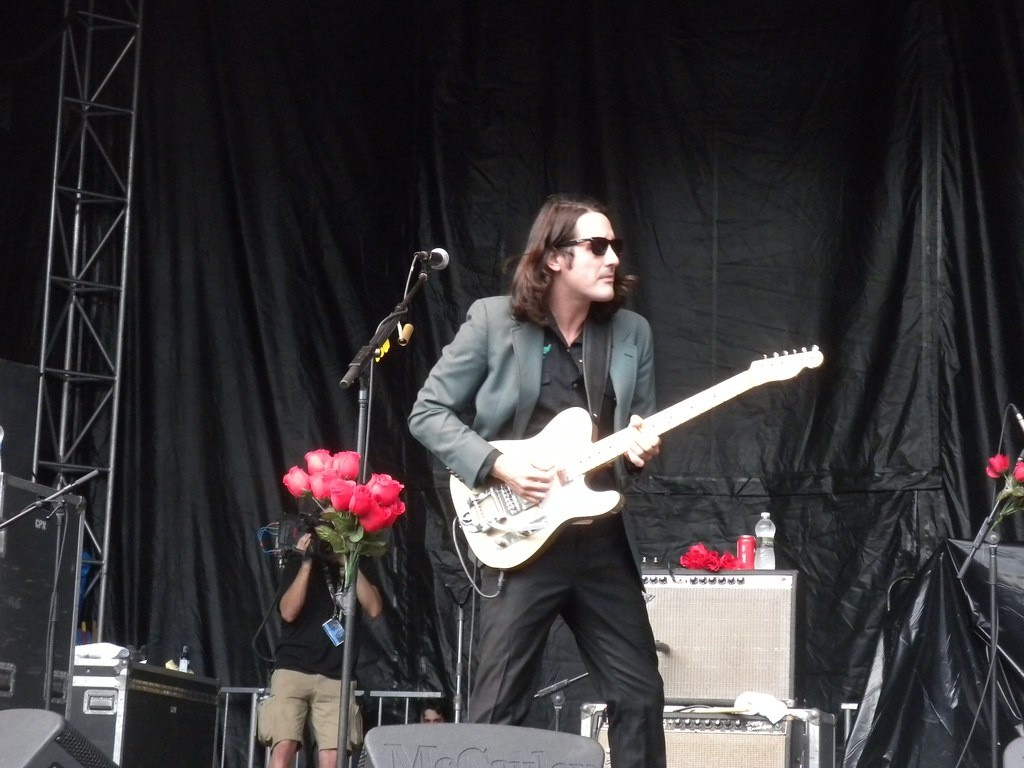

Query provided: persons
[418,696,449,725]
[257,533,383,768]
[407,194,668,767]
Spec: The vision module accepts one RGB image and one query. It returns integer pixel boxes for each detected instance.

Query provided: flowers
[986,452,1024,529]
[677,542,735,573]
[281,447,406,604]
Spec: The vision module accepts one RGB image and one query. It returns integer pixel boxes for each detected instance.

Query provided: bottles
[178,645,190,673]
[753,512,777,570]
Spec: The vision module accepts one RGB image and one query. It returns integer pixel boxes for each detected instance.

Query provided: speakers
[0,708,121,768]
[359,723,606,768]
[640,566,805,708]
[589,711,806,768]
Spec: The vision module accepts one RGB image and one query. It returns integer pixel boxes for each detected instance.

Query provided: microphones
[1010,405,1024,432]
[413,248,450,270]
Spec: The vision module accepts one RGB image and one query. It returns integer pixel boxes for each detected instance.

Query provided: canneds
[737,535,755,570]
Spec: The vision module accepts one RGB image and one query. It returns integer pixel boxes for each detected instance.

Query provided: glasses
[550,237,626,257]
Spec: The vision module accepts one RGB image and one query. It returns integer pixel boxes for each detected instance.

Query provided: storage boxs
[65,660,221,768]
[580,702,836,768]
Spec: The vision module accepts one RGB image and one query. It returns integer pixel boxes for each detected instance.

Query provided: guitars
[447,339,828,579]
